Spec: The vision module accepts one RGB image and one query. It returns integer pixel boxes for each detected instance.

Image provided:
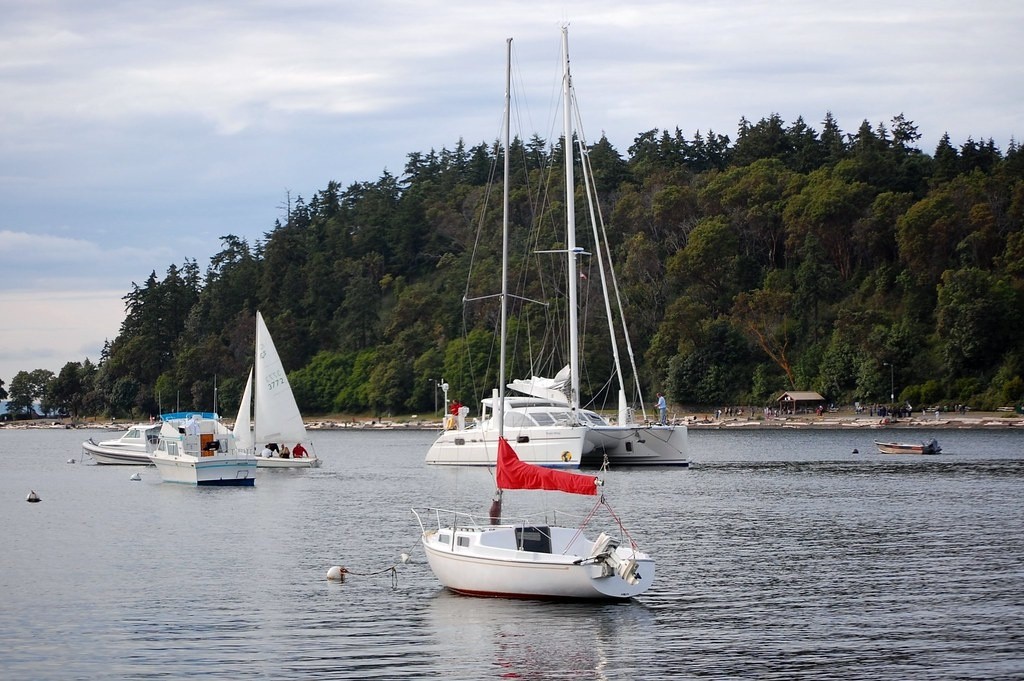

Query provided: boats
[81,374,258,487]
[874,437,943,454]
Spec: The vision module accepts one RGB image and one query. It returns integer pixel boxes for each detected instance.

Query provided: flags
[496,437,597,494]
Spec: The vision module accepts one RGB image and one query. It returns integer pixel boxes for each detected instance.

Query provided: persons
[716,402,834,418]
[655,392,667,426]
[923,401,967,420]
[445,398,462,431]
[855,402,912,417]
[262,443,309,458]
[185,413,200,436]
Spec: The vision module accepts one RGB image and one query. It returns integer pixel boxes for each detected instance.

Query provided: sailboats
[423,13,691,470]
[232,310,323,468]
[410,36,657,604]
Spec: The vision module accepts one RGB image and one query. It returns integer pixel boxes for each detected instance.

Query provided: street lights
[428,378,438,418]
[884,362,894,405]
